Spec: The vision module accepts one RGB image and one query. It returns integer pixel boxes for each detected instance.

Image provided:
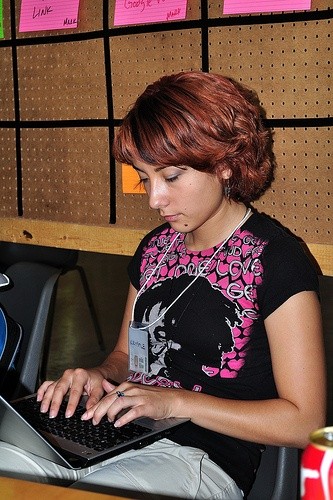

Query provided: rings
[116,391,124,398]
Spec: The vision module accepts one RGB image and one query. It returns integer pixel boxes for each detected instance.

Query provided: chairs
[0,261,104,397]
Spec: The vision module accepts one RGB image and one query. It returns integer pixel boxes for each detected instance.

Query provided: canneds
[300,426,333,500]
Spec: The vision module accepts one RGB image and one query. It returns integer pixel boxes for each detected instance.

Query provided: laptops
[0,393,192,471]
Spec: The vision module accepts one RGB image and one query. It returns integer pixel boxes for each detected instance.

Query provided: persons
[0,71,328,500]
